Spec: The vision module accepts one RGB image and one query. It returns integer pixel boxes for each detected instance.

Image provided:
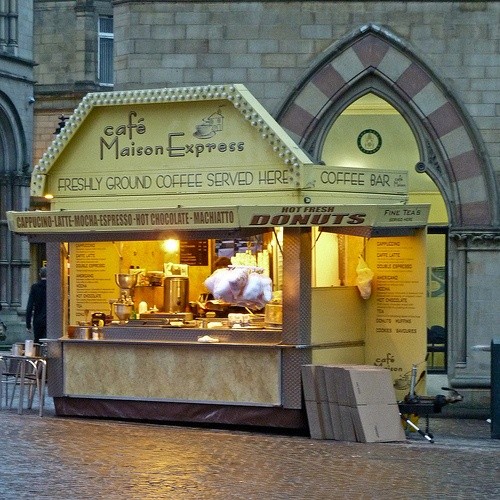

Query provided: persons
[211,257,245,317]
[26,267,47,384]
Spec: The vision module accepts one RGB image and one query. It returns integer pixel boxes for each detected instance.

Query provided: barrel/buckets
[164,277,189,313]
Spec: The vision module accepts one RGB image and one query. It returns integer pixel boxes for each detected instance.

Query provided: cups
[25,340,33,356]
[79,328,90,339]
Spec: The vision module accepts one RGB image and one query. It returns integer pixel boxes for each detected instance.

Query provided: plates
[232,325,282,330]
[171,322,201,328]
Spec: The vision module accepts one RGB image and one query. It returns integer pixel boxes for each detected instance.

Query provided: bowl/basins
[113,303,134,320]
[68,326,93,339]
[13,343,40,357]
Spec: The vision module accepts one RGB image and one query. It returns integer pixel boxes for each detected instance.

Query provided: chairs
[427,325,446,369]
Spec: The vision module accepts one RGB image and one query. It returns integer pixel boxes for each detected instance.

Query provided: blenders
[109,274,137,325]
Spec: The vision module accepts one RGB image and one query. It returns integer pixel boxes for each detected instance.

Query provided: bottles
[91,327,99,340]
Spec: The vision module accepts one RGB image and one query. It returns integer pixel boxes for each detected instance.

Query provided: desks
[0,354,48,418]
